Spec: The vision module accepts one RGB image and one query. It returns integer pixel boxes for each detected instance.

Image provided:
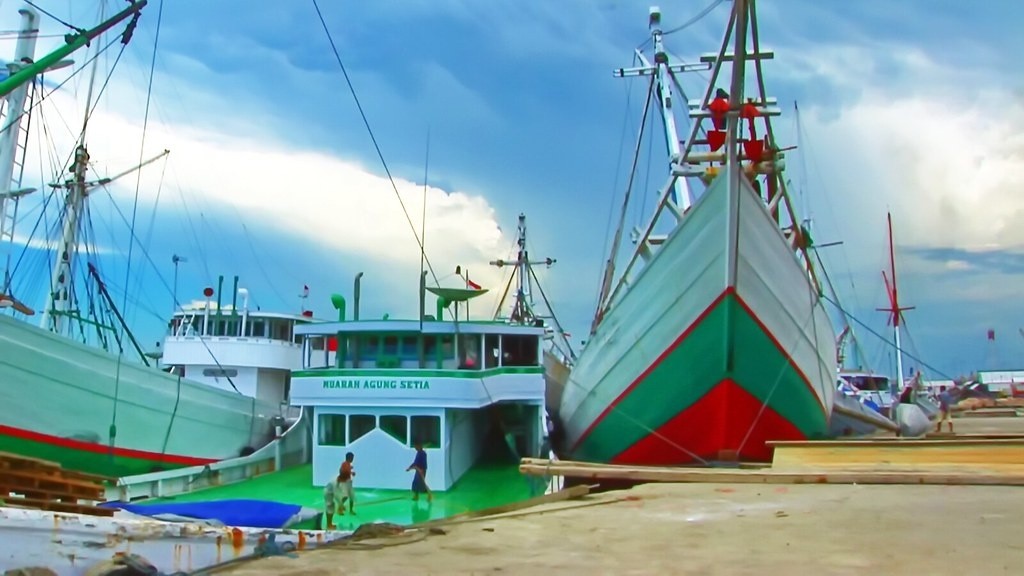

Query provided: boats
[831,369,891,415]
[0,208,563,574]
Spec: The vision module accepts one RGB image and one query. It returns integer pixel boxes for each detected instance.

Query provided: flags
[469,280,481,291]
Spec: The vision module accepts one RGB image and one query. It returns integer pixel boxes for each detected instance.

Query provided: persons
[936,385,953,432]
[325,452,356,528]
[406,440,433,501]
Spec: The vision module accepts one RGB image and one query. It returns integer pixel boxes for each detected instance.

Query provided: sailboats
[0,0,357,512]
[541,0,876,493]
